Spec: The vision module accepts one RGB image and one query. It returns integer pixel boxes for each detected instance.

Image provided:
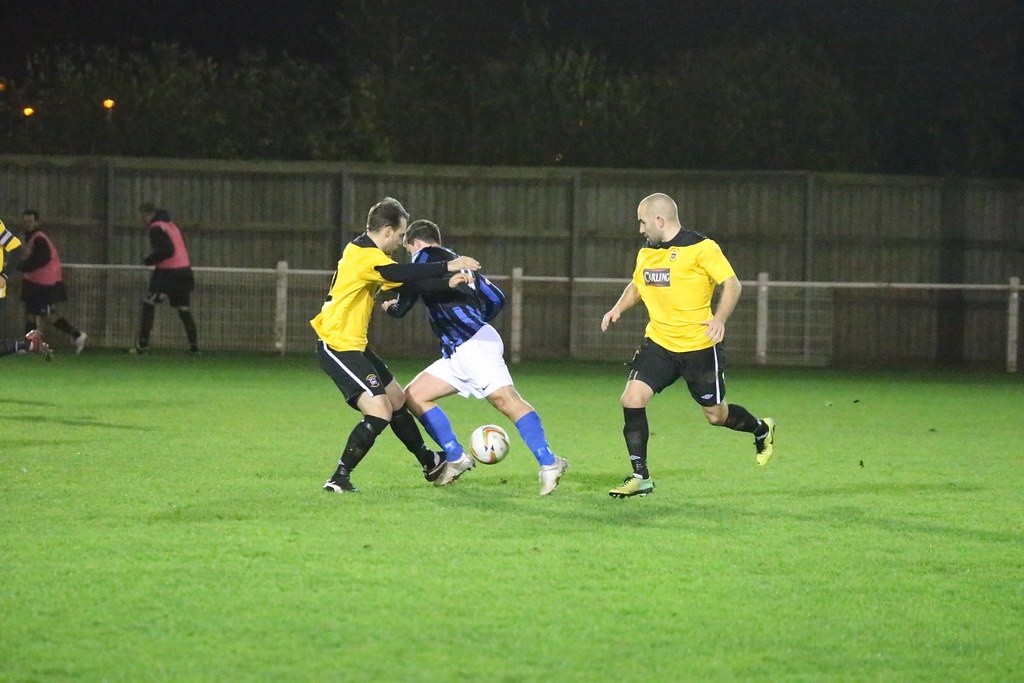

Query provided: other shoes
[123,345,146,356]
[74,331,88,355]
[186,350,202,357]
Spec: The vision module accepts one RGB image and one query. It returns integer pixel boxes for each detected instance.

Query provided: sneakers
[538,455,568,495]
[323,479,359,495]
[433,451,476,487]
[753,417,776,466]
[609,473,654,499]
[422,451,447,482]
[26,329,54,362]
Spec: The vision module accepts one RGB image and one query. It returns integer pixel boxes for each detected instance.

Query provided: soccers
[469,424,510,465]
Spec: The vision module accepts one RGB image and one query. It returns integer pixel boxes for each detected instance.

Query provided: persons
[15,209,87,354]
[601,193,776,499]
[0,220,22,300]
[310,197,481,494]
[381,219,568,496]
[122,202,200,354]
